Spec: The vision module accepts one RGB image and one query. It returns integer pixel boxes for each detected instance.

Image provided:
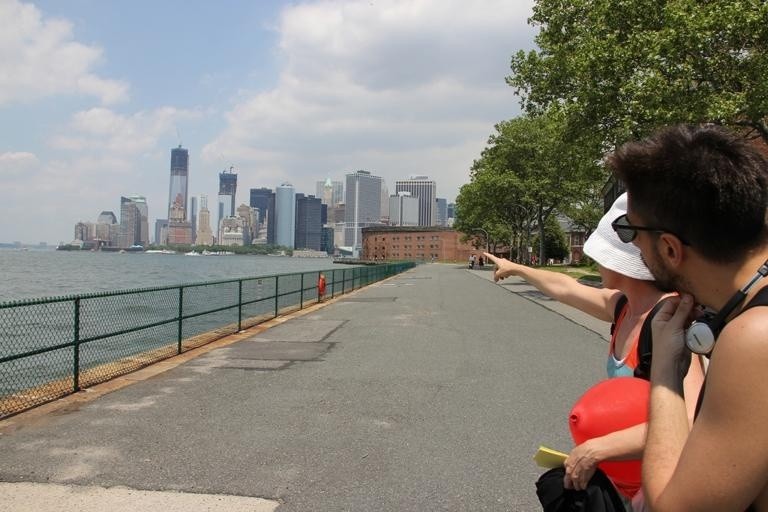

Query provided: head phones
[684,258,768,356]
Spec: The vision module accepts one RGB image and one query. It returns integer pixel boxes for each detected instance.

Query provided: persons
[482,190,706,512]
[607,124,768,512]
[469,254,476,266]
[479,256,484,266]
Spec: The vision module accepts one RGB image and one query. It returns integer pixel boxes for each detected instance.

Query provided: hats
[582,193,656,281]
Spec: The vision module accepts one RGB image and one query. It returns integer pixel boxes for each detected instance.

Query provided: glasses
[611,214,692,246]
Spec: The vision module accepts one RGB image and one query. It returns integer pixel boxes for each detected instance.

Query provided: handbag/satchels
[535,467,626,512]
